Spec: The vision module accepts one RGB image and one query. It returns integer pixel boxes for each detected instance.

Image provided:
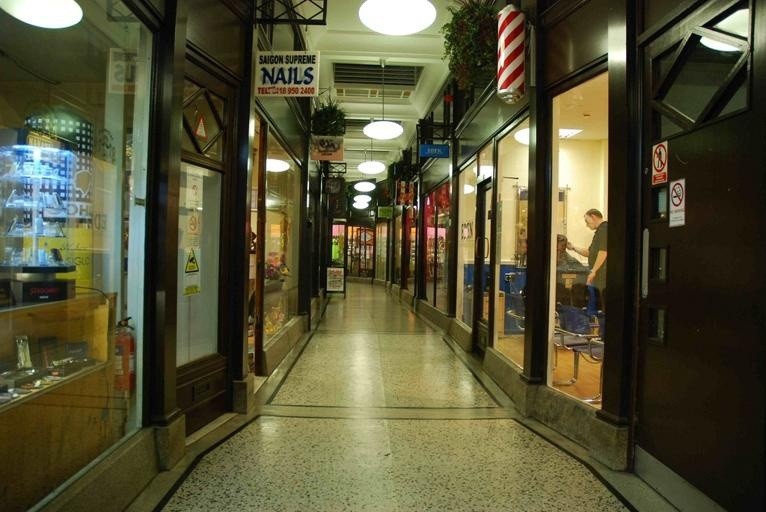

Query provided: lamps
[357,59,404,175]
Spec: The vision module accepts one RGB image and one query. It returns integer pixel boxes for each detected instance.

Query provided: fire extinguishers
[114,317,136,391]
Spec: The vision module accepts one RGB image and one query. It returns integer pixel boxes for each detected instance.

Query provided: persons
[565,208,608,341]
[556,233,586,284]
[518,227,527,267]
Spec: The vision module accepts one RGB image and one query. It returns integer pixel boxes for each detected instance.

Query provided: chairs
[509,297,605,403]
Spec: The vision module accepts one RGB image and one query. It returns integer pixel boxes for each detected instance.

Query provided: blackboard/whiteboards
[325,266,346,293]
